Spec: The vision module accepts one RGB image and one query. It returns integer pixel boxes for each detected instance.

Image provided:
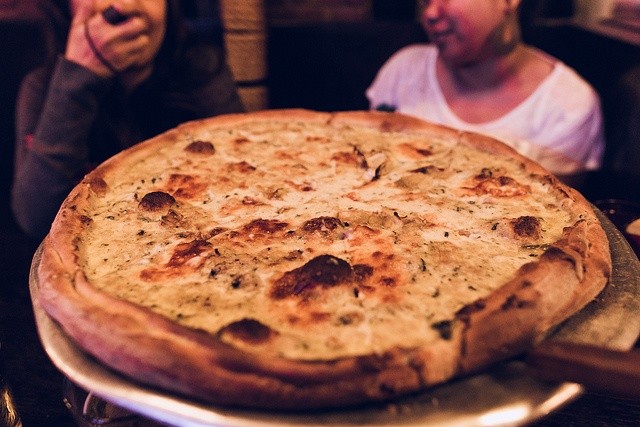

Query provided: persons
[0,0,246,426]
[366,0,601,169]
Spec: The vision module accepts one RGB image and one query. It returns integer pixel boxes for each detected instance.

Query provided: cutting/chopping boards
[29,198,636,426]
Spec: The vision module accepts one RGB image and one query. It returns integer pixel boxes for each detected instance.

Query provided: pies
[37,106,613,414]
